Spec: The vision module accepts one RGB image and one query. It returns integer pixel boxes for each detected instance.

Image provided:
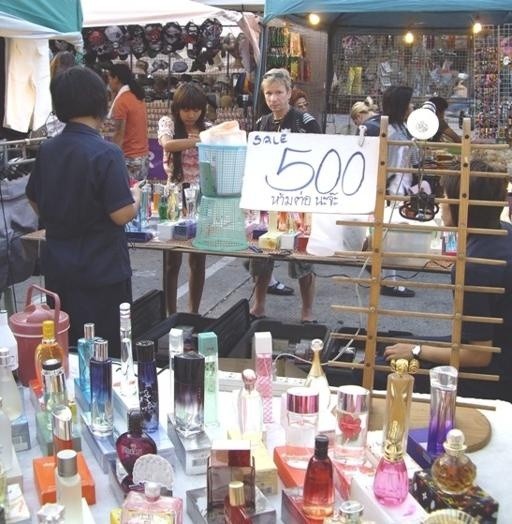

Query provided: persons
[104,61,151,185]
[247,67,322,325]
[48,50,76,78]
[360,158,512,391]
[155,80,216,318]
[378,81,416,297]
[243,260,295,296]
[25,64,143,359]
[285,88,309,114]
[424,95,463,143]
[348,93,383,136]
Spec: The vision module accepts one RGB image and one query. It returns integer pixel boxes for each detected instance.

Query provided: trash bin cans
[192,195,249,251]
[195,143,247,197]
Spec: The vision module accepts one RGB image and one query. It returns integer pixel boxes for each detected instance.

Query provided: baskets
[193,142,250,251]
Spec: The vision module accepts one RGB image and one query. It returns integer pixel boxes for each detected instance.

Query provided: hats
[97,59,113,70]
[82,18,222,62]
[152,60,168,73]
[134,76,252,108]
[135,60,150,74]
[172,62,187,73]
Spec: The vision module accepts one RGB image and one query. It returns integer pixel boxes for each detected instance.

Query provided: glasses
[297,102,310,108]
[263,72,284,79]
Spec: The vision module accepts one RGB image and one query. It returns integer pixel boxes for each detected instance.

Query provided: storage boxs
[323,327,391,389]
[230,318,329,370]
[133,310,215,366]
[383,208,439,267]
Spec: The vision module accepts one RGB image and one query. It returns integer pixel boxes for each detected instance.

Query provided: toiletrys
[246,209,311,234]
[1,300,481,524]
[136,179,201,226]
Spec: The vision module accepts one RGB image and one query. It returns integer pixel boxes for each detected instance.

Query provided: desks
[22,230,453,326]
[0,354,512,524]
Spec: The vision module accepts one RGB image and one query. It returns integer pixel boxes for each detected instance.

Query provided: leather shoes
[267,281,295,295]
[380,284,415,298]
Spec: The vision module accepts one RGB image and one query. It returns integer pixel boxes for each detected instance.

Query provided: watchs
[194,139,201,150]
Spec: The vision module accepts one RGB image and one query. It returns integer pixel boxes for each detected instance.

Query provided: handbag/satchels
[34,245,48,276]
